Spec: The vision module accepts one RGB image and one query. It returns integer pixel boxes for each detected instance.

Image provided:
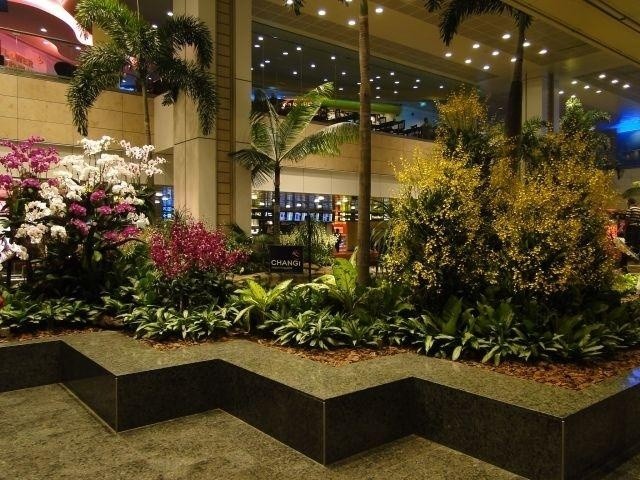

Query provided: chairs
[273,98,435,143]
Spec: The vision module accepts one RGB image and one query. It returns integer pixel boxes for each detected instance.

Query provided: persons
[335,228,340,252]
[421,117,432,135]
